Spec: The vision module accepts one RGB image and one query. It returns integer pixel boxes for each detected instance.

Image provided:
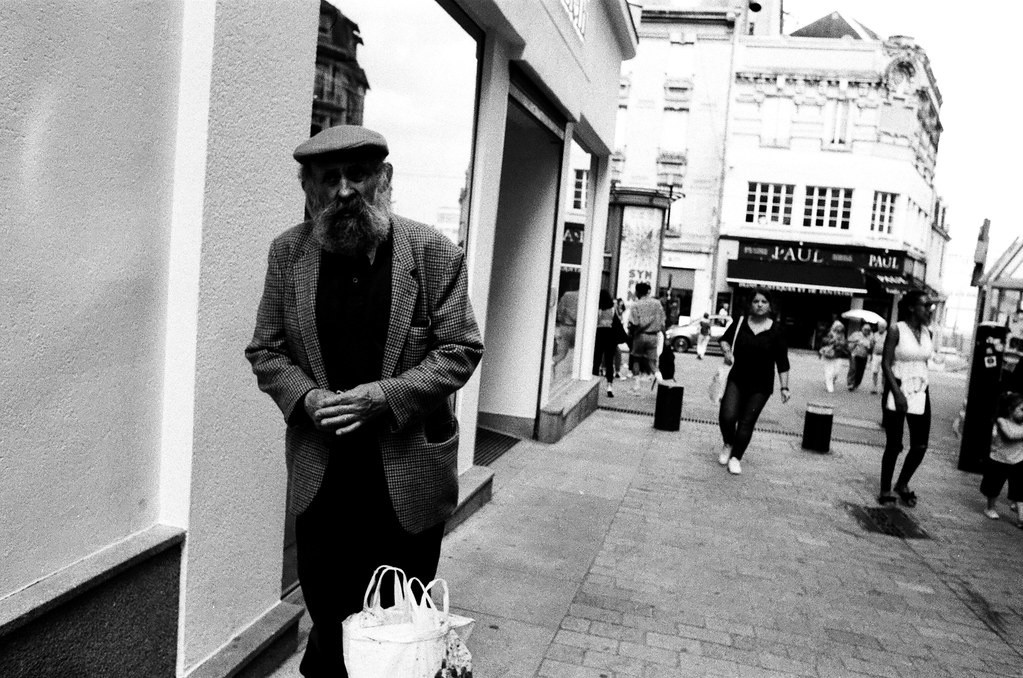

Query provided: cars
[665,315,734,352]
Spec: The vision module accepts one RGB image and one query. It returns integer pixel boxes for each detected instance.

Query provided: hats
[293,124,389,162]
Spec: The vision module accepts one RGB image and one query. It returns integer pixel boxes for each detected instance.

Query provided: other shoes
[983,507,999,520]
[727,457,742,475]
[718,444,732,465]
[607,384,615,398]
[1009,506,1023,528]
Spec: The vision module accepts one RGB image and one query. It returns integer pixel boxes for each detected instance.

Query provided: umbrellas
[843,310,887,330]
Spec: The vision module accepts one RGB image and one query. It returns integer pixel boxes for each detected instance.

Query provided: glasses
[912,302,934,308]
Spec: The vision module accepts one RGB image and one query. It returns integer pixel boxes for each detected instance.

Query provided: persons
[717,287,790,475]
[819,320,887,394]
[880,290,935,506]
[550,289,578,376]
[697,303,729,359]
[592,282,666,398]
[979,393,1023,528]
[246,125,485,678]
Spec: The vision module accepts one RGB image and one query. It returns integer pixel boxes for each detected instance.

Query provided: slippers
[893,485,917,507]
[878,494,897,509]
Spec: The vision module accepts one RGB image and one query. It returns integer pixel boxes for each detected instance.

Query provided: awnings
[870,274,946,303]
[727,277,868,297]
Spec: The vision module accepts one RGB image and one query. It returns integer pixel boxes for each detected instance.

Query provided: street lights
[710,0,762,314]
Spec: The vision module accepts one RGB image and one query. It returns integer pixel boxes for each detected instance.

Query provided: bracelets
[780,386,790,392]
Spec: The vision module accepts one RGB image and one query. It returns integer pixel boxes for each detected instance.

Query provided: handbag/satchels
[340,563,477,678]
[708,357,734,404]
